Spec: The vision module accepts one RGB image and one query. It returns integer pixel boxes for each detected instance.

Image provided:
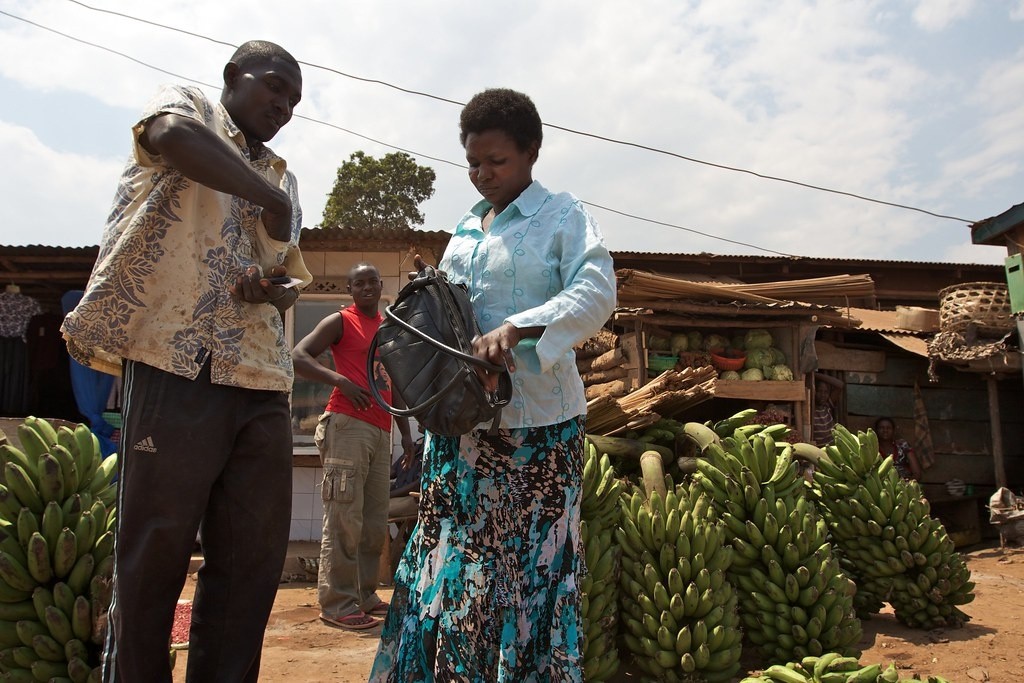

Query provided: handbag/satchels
[367,262,514,438]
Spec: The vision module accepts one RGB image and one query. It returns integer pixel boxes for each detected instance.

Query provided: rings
[503,346,510,352]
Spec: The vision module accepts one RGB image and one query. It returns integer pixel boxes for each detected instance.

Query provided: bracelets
[270,291,288,300]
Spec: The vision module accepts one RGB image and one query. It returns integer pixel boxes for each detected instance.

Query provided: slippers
[319,608,380,629]
[365,601,389,615]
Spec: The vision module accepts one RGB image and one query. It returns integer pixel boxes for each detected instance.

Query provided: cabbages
[649,328,793,382]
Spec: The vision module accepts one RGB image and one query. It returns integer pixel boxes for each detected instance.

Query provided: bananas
[0,416,118,683]
[581,410,975,683]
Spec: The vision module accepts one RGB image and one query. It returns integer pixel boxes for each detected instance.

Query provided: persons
[58,38,312,683]
[368,89,617,683]
[291,264,415,629]
[814,372,844,447]
[388,423,425,518]
[875,417,921,484]
